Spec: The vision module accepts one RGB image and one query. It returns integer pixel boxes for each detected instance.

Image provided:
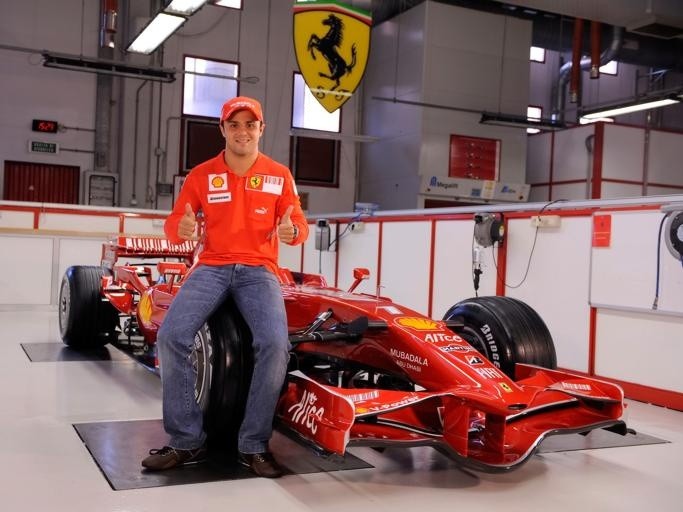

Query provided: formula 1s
[54,211,629,471]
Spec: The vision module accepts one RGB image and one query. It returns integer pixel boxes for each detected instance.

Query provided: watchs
[293,225,298,238]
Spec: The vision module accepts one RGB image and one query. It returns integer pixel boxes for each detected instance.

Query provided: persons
[141,96,310,478]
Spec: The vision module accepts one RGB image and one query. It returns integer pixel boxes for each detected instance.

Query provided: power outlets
[349,222,364,233]
[530,215,561,229]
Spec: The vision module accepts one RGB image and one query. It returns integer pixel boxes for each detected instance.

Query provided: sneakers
[141,445,204,470]
[239,452,283,479]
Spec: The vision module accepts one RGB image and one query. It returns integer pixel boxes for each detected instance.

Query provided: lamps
[480,16,681,131]
[39,0,210,132]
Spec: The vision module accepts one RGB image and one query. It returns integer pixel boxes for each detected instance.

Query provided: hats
[221,97,263,121]
